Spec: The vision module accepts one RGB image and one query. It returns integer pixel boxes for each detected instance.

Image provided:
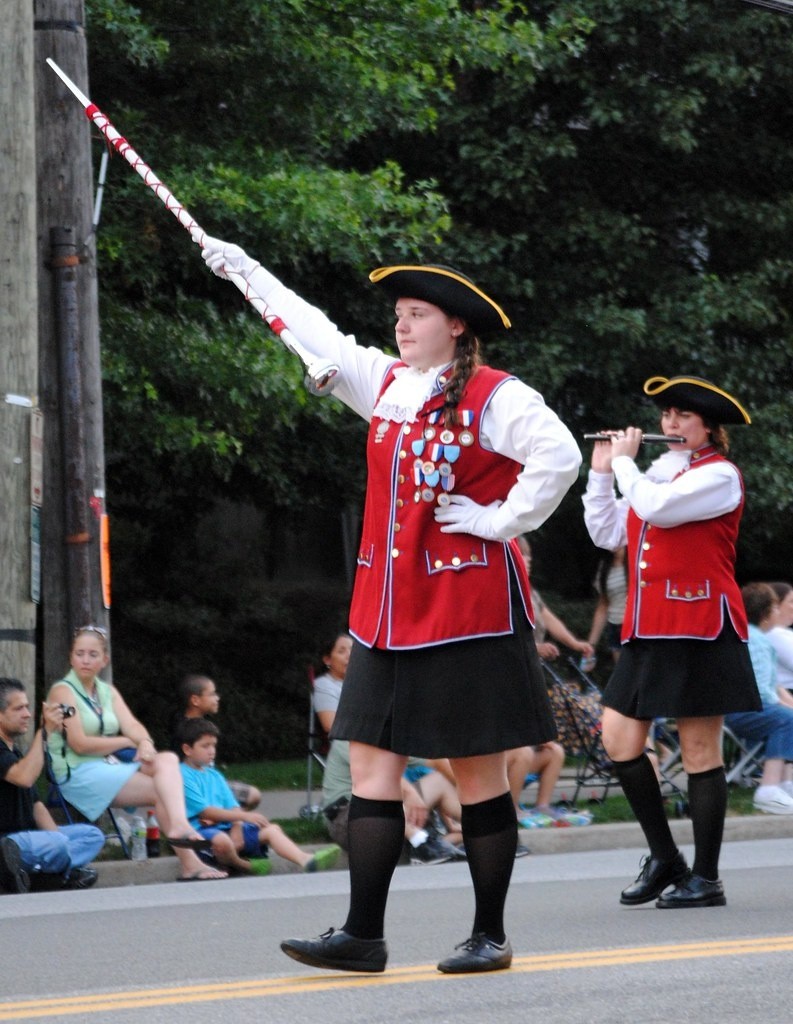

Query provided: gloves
[435,494,507,543]
[191,234,261,283]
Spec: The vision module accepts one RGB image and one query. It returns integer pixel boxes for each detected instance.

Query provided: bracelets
[145,738,155,744]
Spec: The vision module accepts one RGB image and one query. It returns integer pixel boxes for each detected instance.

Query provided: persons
[171,675,261,811]
[178,719,342,873]
[580,545,629,673]
[582,374,763,908]
[42,627,230,882]
[309,536,592,862]
[725,581,793,815]
[0,677,104,892]
[203,235,582,974]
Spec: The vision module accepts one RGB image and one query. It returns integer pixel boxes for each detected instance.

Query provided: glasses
[76,624,109,640]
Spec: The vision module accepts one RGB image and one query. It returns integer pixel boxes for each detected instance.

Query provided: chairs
[298,651,769,830]
[43,717,128,862]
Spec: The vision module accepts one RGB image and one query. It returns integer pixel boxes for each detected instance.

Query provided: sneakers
[0,836,31,895]
[752,789,793,815]
[30,865,98,893]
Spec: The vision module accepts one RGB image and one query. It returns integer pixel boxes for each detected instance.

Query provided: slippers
[303,844,341,873]
[175,865,229,882]
[166,828,213,850]
[245,858,272,876]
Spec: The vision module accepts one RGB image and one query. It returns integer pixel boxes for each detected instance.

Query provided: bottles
[147,810,161,857]
[131,816,141,860]
[136,818,147,862]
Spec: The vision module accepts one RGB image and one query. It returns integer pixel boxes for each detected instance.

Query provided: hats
[643,374,752,428]
[370,263,511,341]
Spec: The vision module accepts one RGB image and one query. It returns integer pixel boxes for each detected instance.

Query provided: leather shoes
[281,926,388,974]
[437,931,512,973]
[619,854,690,905]
[654,869,726,909]
[410,836,455,866]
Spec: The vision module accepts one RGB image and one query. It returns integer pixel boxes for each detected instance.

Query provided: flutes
[581,432,688,443]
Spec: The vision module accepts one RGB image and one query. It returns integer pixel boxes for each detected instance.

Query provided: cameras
[57,703,76,719]
